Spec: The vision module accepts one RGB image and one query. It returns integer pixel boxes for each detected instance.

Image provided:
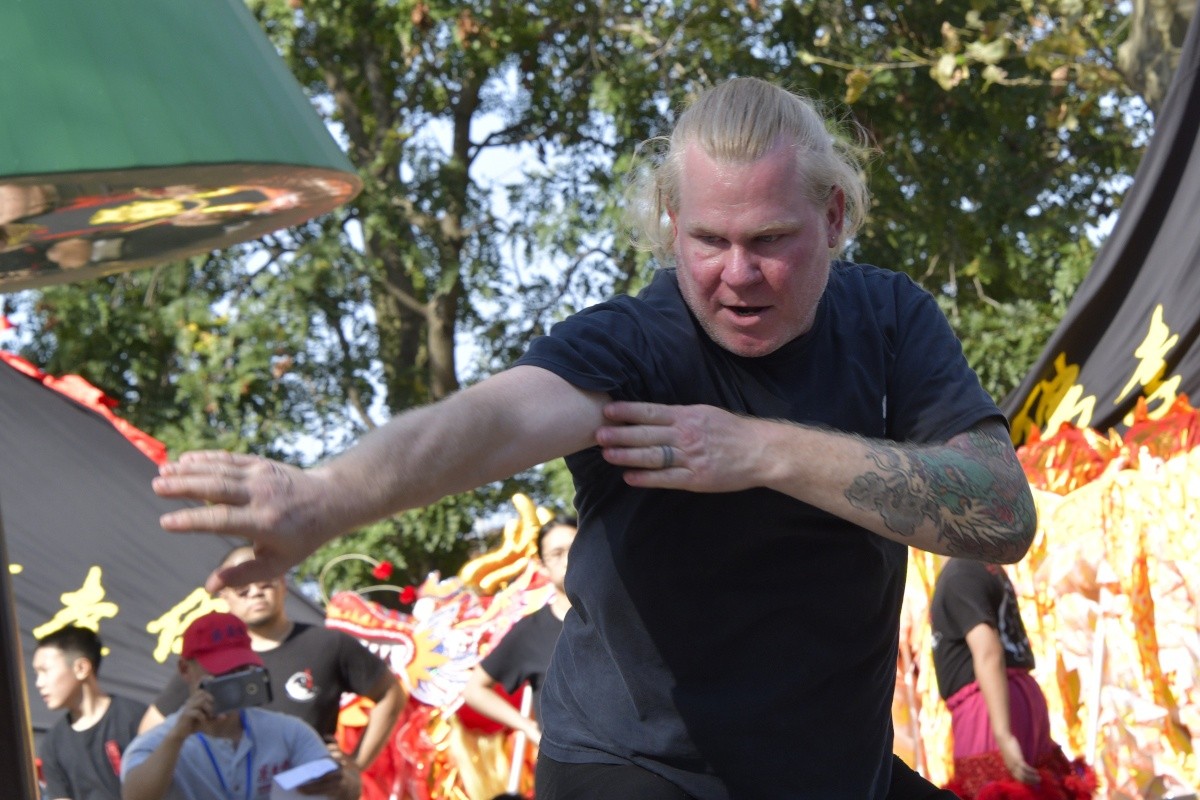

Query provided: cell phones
[204,668,271,715]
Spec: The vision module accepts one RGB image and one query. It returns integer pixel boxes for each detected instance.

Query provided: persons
[29,545,406,800]
[463,513,579,745]
[151,75,1036,800]
[930,554,1098,800]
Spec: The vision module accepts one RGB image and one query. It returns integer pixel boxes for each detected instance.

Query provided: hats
[182,609,266,677]
